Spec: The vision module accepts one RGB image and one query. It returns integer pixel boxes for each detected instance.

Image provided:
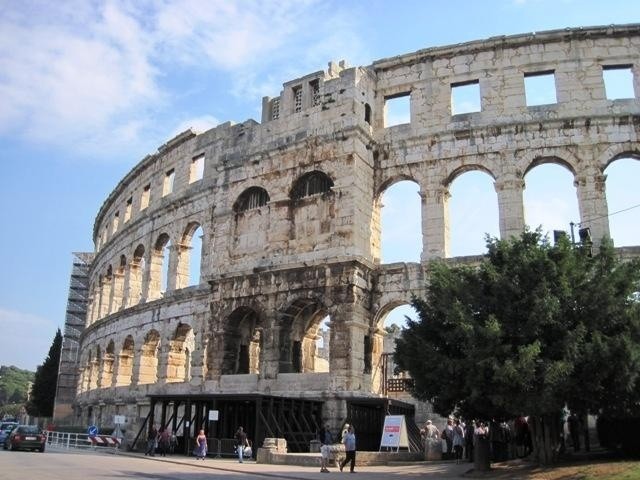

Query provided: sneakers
[321,469,330,473]
[339,465,357,473]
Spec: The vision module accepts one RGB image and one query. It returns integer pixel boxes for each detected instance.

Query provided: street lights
[554,221,593,258]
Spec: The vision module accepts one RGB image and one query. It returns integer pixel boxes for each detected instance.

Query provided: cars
[0,415,47,452]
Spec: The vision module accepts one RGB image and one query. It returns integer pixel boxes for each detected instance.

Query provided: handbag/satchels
[441,430,446,438]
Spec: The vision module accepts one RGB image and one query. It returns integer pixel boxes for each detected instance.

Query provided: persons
[420,425,428,450]
[441,417,568,465]
[319,423,330,473]
[568,413,581,450]
[170,430,179,454]
[233,426,251,463]
[192,429,207,460]
[425,420,439,439]
[144,424,159,457]
[159,427,172,457]
[342,423,349,438]
[339,425,358,474]
